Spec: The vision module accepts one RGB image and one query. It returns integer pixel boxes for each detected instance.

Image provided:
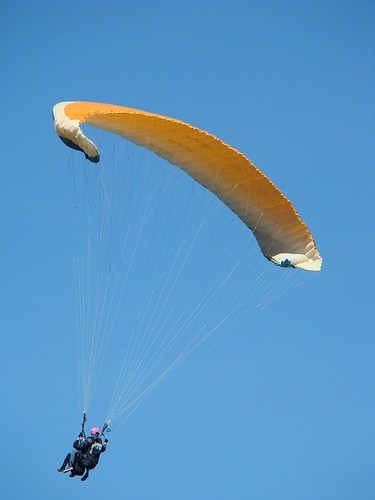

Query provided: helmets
[90,428,99,434]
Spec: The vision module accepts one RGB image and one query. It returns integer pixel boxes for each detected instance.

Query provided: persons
[63,427,107,477]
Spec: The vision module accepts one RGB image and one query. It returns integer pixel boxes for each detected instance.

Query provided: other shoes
[69,473,75,477]
[63,467,73,472]
[57,467,64,471]
[81,474,88,481]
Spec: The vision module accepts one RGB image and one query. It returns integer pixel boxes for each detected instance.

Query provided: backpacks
[82,437,103,468]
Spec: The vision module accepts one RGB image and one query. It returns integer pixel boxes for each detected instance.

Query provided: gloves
[105,439,108,443]
